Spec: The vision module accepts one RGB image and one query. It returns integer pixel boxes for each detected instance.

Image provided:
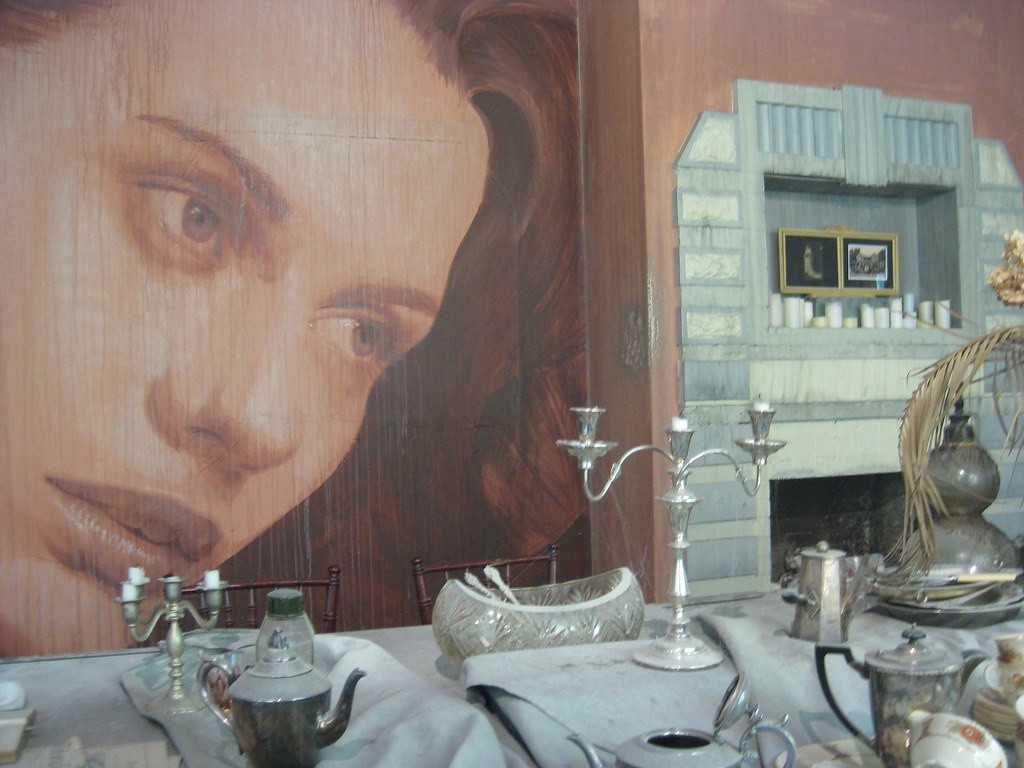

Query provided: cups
[972,632,1024,767]
[768,289,952,332]
[905,711,1010,767]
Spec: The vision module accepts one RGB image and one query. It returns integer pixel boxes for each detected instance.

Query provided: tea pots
[566,671,799,768]
[816,621,990,767]
[780,539,871,646]
[196,630,367,768]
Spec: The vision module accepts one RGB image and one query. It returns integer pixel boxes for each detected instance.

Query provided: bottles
[257,586,315,664]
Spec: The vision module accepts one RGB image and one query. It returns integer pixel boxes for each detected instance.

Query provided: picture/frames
[777,224,901,298]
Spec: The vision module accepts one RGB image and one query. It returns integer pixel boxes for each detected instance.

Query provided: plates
[870,562,1023,627]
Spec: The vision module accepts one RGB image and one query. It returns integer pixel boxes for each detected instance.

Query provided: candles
[662,409,694,461]
[768,290,953,331]
[196,567,232,617]
[745,393,777,442]
[120,564,152,587]
[114,579,146,629]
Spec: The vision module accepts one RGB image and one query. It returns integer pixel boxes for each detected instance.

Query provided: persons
[0,0,594,666]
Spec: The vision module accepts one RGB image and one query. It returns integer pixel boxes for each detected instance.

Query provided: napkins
[120,631,507,768]
[456,609,879,768]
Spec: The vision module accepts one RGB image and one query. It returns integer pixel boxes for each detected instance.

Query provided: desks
[0,585,1024,768]
